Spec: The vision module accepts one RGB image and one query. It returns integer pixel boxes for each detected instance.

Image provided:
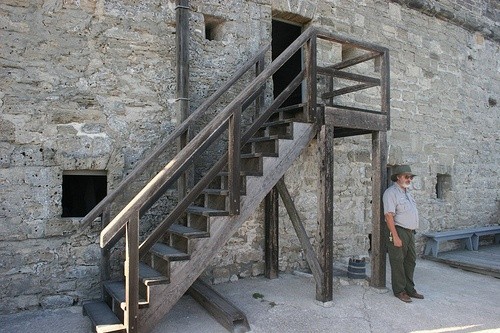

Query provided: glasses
[403,175,414,180]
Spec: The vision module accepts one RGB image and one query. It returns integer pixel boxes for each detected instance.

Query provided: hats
[390,165,416,181]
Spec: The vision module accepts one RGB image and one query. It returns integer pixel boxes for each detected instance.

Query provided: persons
[382,165,424,302]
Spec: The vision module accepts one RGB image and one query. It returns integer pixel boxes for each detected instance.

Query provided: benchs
[423,225,500,257]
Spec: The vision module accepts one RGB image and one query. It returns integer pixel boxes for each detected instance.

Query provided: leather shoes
[411,293,424,299]
[397,292,412,302]
